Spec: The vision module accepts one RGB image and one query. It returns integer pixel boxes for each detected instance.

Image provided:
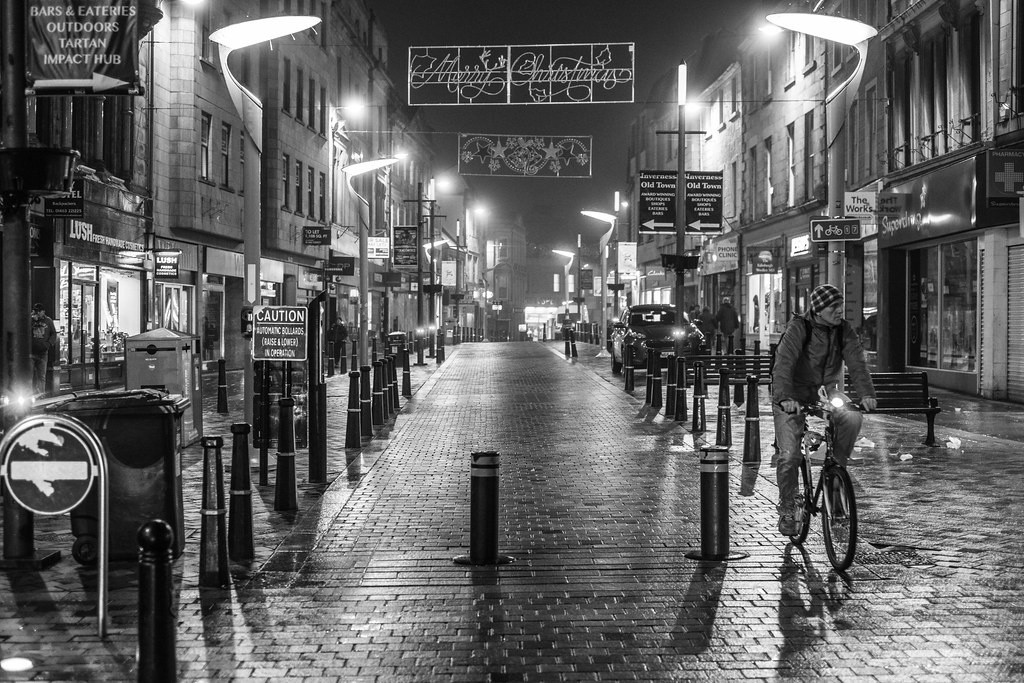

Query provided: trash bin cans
[45,388,192,565]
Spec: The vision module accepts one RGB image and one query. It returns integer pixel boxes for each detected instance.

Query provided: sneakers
[835,515,850,530]
[776,501,798,536]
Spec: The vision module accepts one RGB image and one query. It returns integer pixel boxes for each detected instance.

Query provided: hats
[809,284,844,314]
[722,297,730,304]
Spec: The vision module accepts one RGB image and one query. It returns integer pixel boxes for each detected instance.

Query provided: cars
[610,303,707,373]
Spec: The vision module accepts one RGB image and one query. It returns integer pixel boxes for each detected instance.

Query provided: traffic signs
[23,0,140,96]
[683,171,725,234]
[639,169,679,235]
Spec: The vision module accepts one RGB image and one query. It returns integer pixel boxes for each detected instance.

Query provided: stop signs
[3,428,94,516]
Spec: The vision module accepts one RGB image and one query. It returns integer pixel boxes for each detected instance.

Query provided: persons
[866,315,879,349]
[771,284,878,536]
[333,317,348,368]
[753,286,780,332]
[31,304,57,399]
[202,316,214,361]
[948,296,963,350]
[688,305,718,351]
[393,316,398,332]
[929,328,936,343]
[716,299,739,355]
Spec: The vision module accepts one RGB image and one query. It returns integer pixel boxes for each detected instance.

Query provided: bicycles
[780,400,868,572]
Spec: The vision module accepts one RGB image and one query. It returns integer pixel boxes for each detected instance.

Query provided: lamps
[921,137,930,145]
[77,164,96,176]
[958,118,971,129]
[333,119,345,133]
[108,175,126,185]
[894,148,903,153]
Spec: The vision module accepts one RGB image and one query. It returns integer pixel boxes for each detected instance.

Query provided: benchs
[842,370,941,448]
[685,353,774,406]
